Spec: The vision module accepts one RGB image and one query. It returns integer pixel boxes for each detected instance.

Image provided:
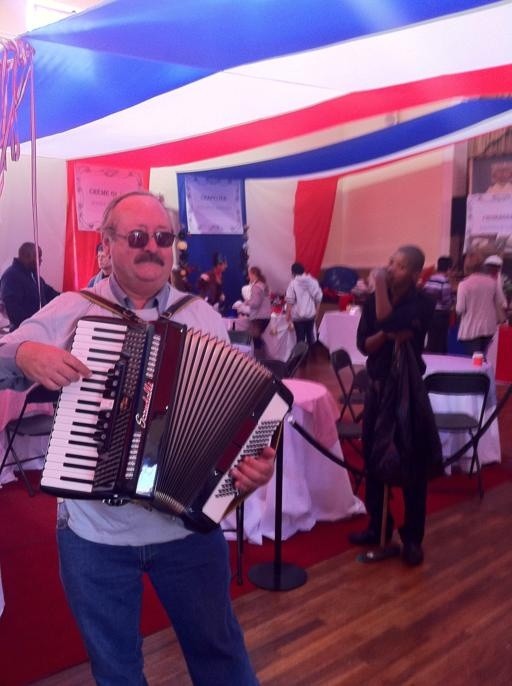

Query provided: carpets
[1,383,512,686]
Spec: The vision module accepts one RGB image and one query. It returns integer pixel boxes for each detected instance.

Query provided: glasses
[115,230,175,249]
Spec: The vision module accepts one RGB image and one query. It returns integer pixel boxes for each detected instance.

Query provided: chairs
[217,301,511,549]
[1,300,57,491]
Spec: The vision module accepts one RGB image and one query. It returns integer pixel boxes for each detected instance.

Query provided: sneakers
[348,529,379,544]
[403,544,424,565]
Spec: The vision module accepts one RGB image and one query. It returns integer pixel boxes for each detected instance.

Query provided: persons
[1,242,62,390]
[198,259,228,316]
[245,267,272,367]
[87,242,112,289]
[285,263,324,345]
[348,243,445,564]
[424,251,512,359]
[1,192,277,685]
